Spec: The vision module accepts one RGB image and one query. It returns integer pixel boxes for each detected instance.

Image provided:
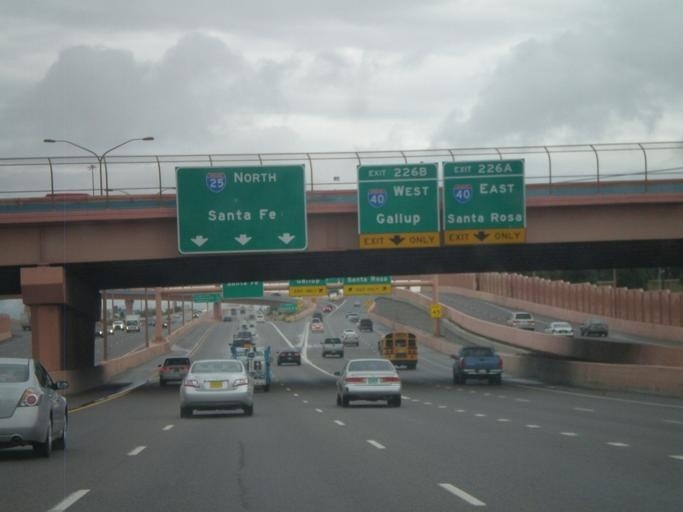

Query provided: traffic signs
[175,164,309,255]
[193,275,392,304]
[356,159,527,249]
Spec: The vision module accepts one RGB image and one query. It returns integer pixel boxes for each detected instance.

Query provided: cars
[543,321,574,337]
[310,304,374,359]
[95,309,301,418]
[333,358,402,408]
[0,357,68,457]
[578,319,609,338]
[506,311,536,330]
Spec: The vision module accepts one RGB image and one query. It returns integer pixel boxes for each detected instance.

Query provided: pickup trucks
[451,345,504,385]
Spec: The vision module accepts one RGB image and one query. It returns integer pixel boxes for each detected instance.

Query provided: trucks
[378,332,418,369]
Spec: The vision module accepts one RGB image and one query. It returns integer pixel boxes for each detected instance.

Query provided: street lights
[43,137,155,362]
